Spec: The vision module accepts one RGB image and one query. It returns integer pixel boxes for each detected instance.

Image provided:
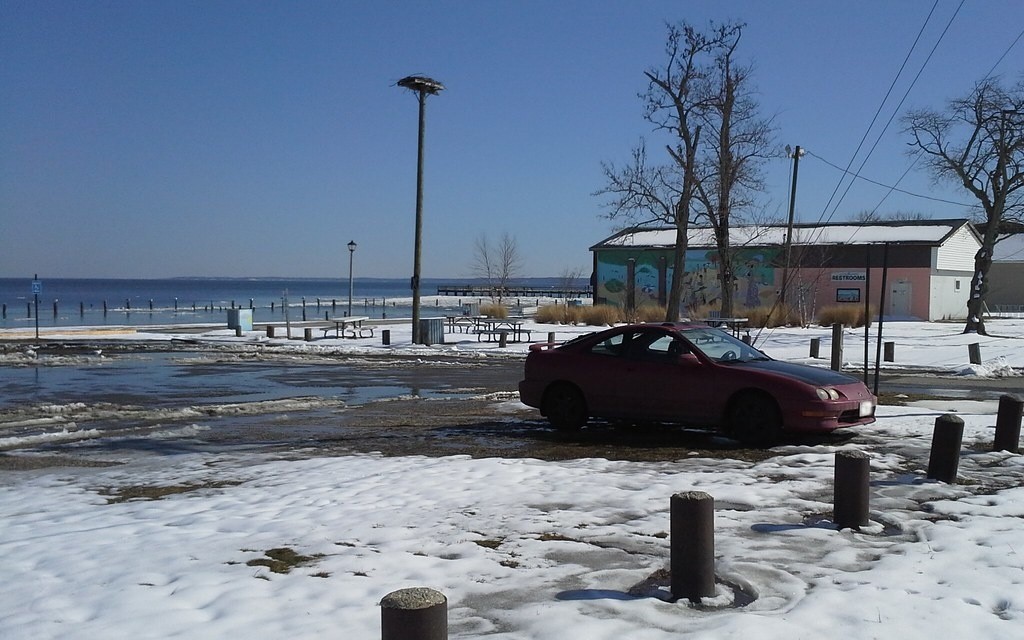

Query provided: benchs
[476,323,491,327]
[347,325,378,332]
[474,329,513,335]
[320,325,343,331]
[501,328,531,334]
[444,323,472,326]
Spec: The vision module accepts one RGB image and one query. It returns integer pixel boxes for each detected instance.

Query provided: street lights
[397,77,444,343]
[346,241,358,315]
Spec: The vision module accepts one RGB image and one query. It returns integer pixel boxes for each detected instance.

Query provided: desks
[696,316,748,342]
[444,315,532,343]
[324,316,374,339]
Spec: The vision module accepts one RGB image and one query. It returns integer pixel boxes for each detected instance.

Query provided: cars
[518,321,877,440]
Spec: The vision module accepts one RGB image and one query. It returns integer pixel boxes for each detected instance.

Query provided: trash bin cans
[227,308,253,331]
[420,317,447,344]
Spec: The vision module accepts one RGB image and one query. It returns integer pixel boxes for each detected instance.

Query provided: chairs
[668,341,691,357]
